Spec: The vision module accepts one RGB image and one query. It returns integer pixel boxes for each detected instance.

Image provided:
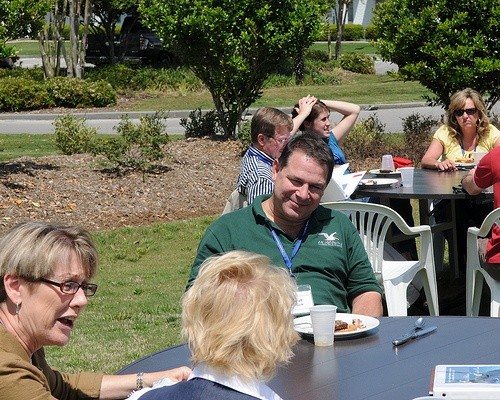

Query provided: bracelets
[135,372,144,390]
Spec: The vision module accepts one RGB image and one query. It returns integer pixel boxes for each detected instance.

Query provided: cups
[400,167,414,187]
[474,151,487,166]
[381,155,397,172]
[403,187,414,194]
[309,305,337,347]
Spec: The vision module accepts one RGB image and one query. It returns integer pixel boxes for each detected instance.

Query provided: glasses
[454,107,478,116]
[268,136,287,144]
[20,275,97,297]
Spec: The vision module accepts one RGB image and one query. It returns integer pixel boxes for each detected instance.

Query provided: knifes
[392,326,437,345]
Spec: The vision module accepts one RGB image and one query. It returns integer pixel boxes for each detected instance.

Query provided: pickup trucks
[85,16,175,69]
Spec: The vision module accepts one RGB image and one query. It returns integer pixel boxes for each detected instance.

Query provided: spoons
[401,318,425,339]
[293,320,348,330]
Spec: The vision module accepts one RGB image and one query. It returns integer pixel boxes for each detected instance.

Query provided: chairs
[221,188,446,317]
[466,208,500,318]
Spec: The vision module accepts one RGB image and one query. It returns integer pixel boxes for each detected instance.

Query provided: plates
[368,168,403,177]
[357,178,399,188]
[455,162,475,168]
[293,313,380,338]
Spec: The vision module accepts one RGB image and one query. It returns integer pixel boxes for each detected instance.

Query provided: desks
[355,166,494,291]
[117,317,500,399]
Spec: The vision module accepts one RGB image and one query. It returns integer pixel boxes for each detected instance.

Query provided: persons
[183,134,383,319]
[0,219,192,400]
[130,251,300,400]
[288,95,413,261]
[420,88,500,272]
[461,144,500,283]
[234,106,295,208]
